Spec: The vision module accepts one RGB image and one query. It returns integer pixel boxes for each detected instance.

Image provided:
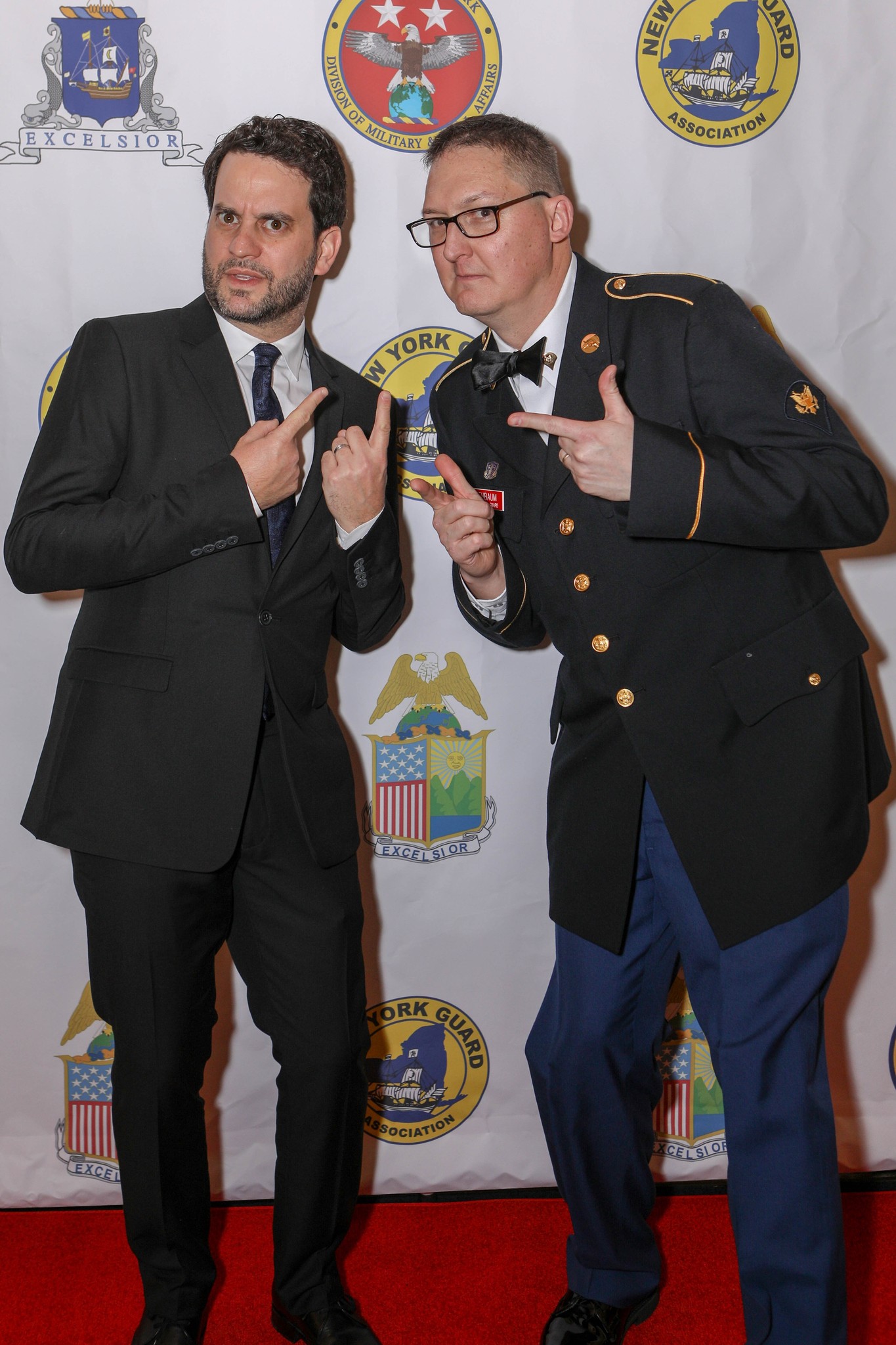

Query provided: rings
[562,453,569,462]
[333,444,350,458]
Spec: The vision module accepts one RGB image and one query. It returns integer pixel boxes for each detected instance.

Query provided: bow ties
[471,337,546,392]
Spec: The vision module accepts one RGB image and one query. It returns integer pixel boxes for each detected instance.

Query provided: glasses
[406,191,552,249]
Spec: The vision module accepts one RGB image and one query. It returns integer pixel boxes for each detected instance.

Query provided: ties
[252,344,296,569]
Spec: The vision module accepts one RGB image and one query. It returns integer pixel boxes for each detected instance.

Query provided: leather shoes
[540,1283,660,1345]
[131,1304,208,1345]
[271,1291,382,1345]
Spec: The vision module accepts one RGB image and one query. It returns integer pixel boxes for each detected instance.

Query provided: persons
[3,116,407,1345]
[410,117,892,1345]
